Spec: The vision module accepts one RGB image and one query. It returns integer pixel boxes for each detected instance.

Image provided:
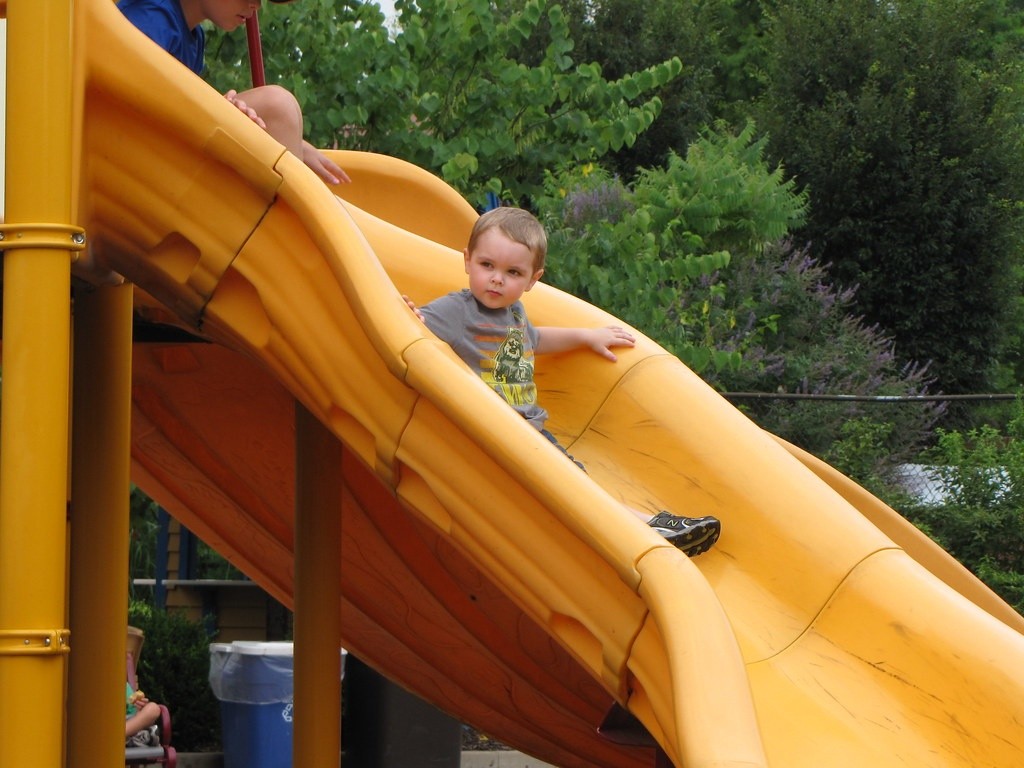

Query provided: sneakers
[645,511,721,557]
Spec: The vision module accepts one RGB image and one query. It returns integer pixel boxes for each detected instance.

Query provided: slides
[76,2,1020,768]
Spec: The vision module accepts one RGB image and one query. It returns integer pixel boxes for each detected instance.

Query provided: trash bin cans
[208,641,348,767]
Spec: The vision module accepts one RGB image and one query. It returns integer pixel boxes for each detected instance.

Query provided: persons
[118,1,350,185]
[126,681,161,748]
[400,206,722,558]
